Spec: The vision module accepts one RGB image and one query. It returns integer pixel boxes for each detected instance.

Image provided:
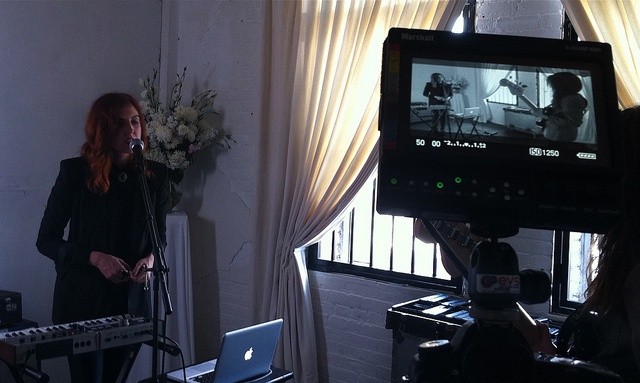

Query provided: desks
[137,356,294,382]
[0,318,42,372]
[450,114,482,140]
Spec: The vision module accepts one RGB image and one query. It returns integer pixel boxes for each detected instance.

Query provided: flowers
[134,65,237,210]
[447,76,469,94]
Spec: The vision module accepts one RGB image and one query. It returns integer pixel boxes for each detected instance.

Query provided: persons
[531,105,640,373]
[414,217,485,277]
[36,92,171,383]
[535,72,587,142]
[423,73,453,132]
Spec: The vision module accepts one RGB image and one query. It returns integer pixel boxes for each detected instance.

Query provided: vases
[161,181,182,214]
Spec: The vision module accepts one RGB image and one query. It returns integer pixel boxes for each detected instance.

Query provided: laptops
[457,106,480,119]
[166,318,285,383]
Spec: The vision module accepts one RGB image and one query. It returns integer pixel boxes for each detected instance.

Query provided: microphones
[128,138,145,174]
